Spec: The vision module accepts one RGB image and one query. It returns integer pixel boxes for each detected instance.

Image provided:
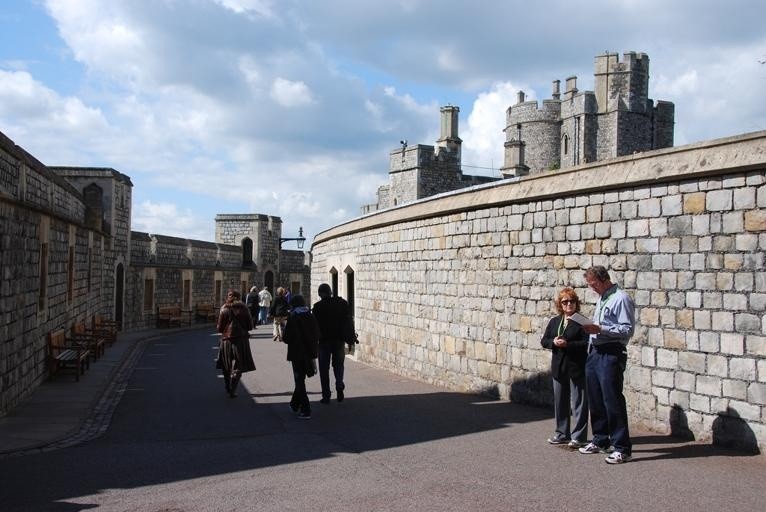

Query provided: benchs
[156,303,192,329]
[92,312,121,347]
[48,321,90,382]
[71,319,105,363]
[196,303,220,323]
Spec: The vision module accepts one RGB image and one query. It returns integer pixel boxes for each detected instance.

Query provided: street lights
[279,227,306,250]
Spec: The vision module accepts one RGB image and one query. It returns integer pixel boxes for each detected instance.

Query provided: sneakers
[578,440,612,454]
[605,450,634,464]
[296,412,312,419]
[548,433,572,445]
[287,402,299,414]
[567,439,587,449]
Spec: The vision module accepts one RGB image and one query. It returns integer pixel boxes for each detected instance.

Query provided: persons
[270,287,288,342]
[283,295,317,420]
[313,284,359,404]
[259,287,272,324]
[215,288,257,400]
[540,289,591,448]
[246,286,261,330]
[283,288,291,304]
[579,265,635,464]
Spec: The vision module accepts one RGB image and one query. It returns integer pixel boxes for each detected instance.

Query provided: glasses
[560,299,577,305]
